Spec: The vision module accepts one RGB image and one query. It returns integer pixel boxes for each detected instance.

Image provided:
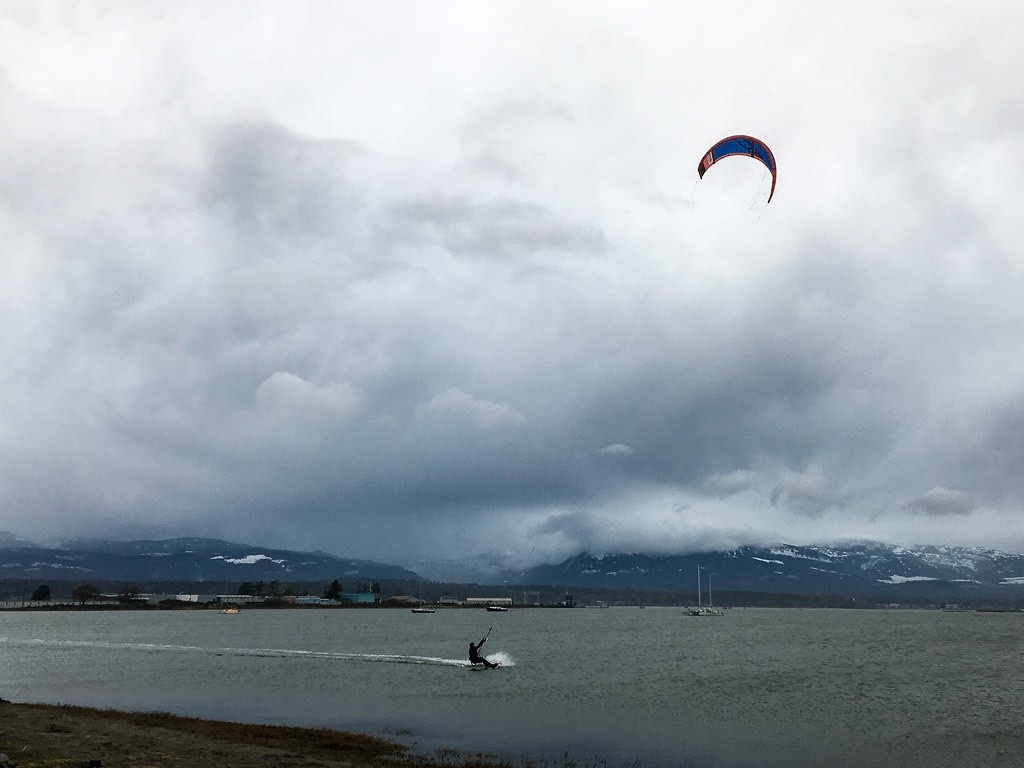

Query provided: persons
[468,638,499,669]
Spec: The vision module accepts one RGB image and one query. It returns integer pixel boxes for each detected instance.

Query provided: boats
[411,609,436,613]
[218,609,244,614]
[486,606,508,611]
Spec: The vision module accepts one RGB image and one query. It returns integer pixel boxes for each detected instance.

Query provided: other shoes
[493,663,497,669]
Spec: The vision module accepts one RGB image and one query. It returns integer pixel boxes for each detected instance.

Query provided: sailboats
[682,564,723,617]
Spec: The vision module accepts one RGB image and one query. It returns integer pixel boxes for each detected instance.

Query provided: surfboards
[482,661,502,669]
